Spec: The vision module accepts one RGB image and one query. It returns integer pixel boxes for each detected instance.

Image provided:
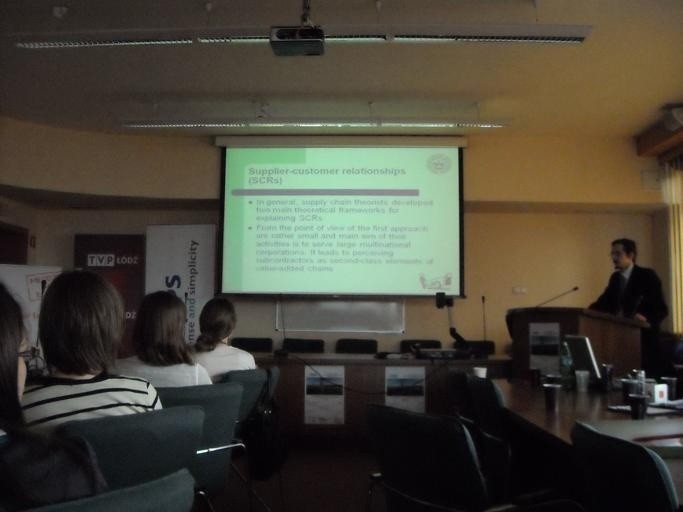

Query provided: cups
[472,367,487,379]
[575,370,590,394]
[661,377,677,400]
[620,378,638,405]
[542,383,562,412]
[547,373,562,384]
[628,393,650,420]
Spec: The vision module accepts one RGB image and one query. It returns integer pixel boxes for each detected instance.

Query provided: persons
[19,270,164,444]
[587,238,669,385]
[1,281,109,511]
[106,290,214,389]
[183,295,259,379]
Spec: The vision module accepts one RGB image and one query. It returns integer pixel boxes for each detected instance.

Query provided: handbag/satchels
[247,397,281,438]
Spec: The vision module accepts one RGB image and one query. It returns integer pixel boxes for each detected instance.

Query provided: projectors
[269,26,325,55]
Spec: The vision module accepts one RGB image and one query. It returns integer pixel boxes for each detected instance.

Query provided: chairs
[335,338,378,355]
[27,468,194,512]
[212,367,267,444]
[154,382,276,511]
[281,338,326,354]
[453,340,495,360]
[231,337,274,355]
[361,403,587,512]
[400,339,442,355]
[449,367,505,421]
[571,422,680,512]
[451,406,514,466]
[57,405,217,511]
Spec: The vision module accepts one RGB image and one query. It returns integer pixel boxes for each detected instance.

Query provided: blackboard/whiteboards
[275,297,405,334]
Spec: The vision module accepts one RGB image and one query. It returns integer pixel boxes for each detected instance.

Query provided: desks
[480,377,683,507]
[253,359,517,450]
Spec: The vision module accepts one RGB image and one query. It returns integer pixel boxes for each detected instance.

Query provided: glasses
[17,345,40,364]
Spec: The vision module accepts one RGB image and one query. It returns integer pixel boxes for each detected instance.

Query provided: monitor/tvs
[566,334,607,384]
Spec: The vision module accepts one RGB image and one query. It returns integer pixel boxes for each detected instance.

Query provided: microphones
[535,285,580,306]
[477,292,491,342]
